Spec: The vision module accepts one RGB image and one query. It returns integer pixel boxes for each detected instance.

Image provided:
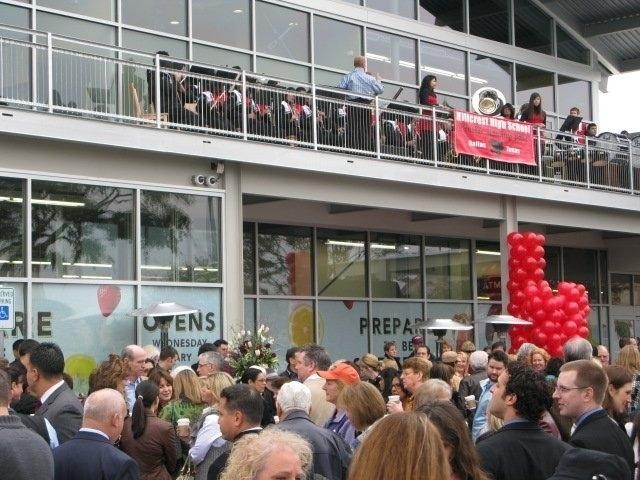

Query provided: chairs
[129,82,167,129]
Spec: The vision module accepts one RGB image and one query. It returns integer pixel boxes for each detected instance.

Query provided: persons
[518,92,547,180]
[419,75,444,165]
[146,54,347,153]
[379,107,423,164]
[438,111,507,176]
[554,107,583,162]
[338,56,384,157]
[0,337,640,480]
[500,103,518,177]
[578,123,597,161]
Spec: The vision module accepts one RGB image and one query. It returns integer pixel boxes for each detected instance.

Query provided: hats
[442,350,459,363]
[411,336,425,345]
[312,362,359,385]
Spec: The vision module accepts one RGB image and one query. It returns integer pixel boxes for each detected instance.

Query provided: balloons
[507,232,590,357]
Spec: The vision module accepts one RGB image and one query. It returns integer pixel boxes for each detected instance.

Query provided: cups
[387,394,403,404]
[465,396,477,410]
[178,417,190,439]
[274,415,279,423]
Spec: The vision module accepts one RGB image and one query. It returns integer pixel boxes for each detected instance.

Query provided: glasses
[196,362,210,367]
[554,383,587,394]
